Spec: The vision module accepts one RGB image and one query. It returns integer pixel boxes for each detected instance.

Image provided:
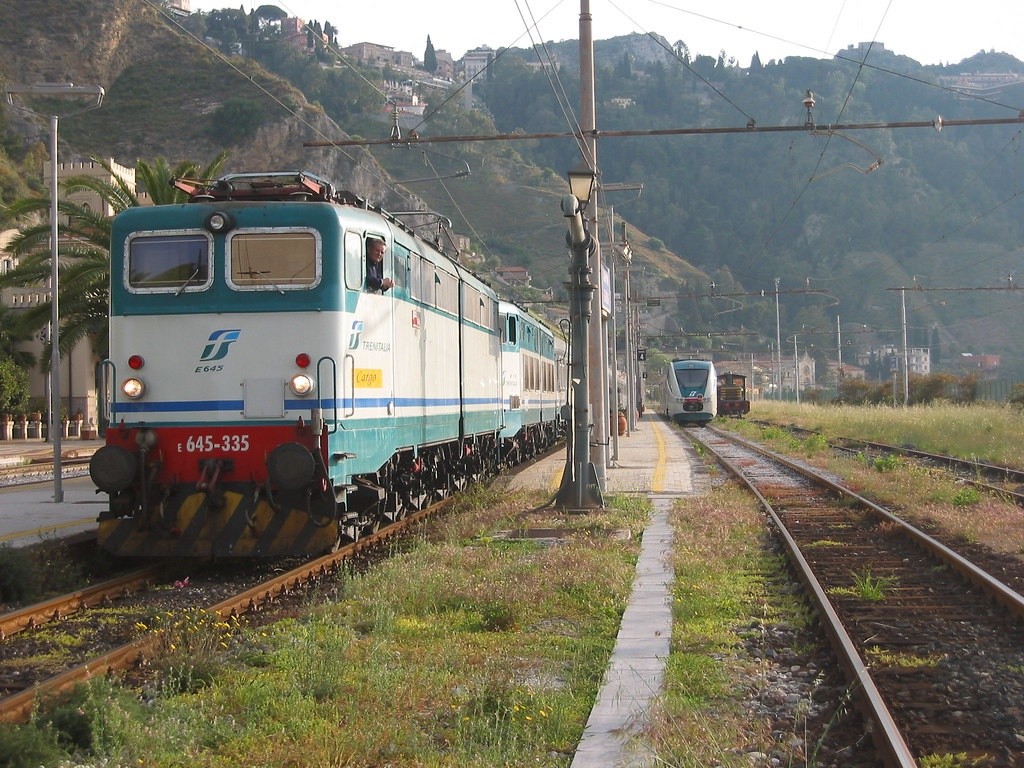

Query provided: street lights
[4,81,106,504]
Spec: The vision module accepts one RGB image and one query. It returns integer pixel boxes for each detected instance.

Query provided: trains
[659,359,717,425]
[88,171,637,558]
[716,372,750,418]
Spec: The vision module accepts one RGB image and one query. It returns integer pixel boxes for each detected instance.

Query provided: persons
[365,237,394,292]
